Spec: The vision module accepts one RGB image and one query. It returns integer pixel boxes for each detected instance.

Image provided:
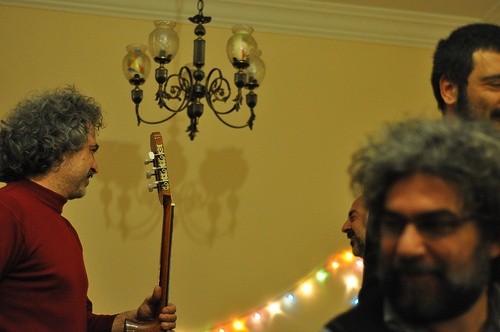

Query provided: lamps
[123,0,265,141]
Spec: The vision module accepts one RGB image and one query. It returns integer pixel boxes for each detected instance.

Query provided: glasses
[382,210,483,235]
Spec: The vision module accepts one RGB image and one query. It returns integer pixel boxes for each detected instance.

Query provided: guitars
[124,131,177,332]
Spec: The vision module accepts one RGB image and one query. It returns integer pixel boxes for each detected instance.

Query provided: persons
[0,80,177,332]
[321,23,500,332]
